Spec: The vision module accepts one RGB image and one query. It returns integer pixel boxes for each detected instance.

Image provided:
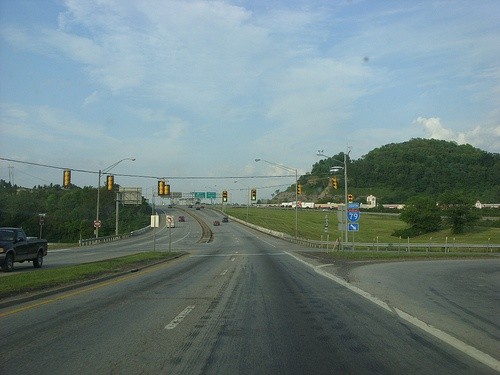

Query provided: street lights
[96,157,136,238]
[315,148,349,243]
[253,158,299,237]
[234,181,249,218]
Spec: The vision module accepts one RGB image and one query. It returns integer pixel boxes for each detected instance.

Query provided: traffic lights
[348,193,353,203]
[251,188,257,201]
[297,184,301,195]
[223,190,227,203]
[332,177,337,190]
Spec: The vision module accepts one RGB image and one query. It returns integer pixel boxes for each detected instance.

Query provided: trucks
[165,215,175,228]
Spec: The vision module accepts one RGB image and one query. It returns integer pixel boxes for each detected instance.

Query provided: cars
[200,205,204,208]
[178,215,185,222]
[188,205,192,208]
[214,220,220,225]
[222,216,228,222]
[196,207,200,210]
[168,205,172,208]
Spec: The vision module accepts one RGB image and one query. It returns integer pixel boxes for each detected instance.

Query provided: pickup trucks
[0,227,49,273]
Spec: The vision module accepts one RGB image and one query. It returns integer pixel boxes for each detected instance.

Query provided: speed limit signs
[347,210,360,223]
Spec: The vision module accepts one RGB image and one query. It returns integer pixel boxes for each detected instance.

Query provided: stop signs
[94,220,101,228]
